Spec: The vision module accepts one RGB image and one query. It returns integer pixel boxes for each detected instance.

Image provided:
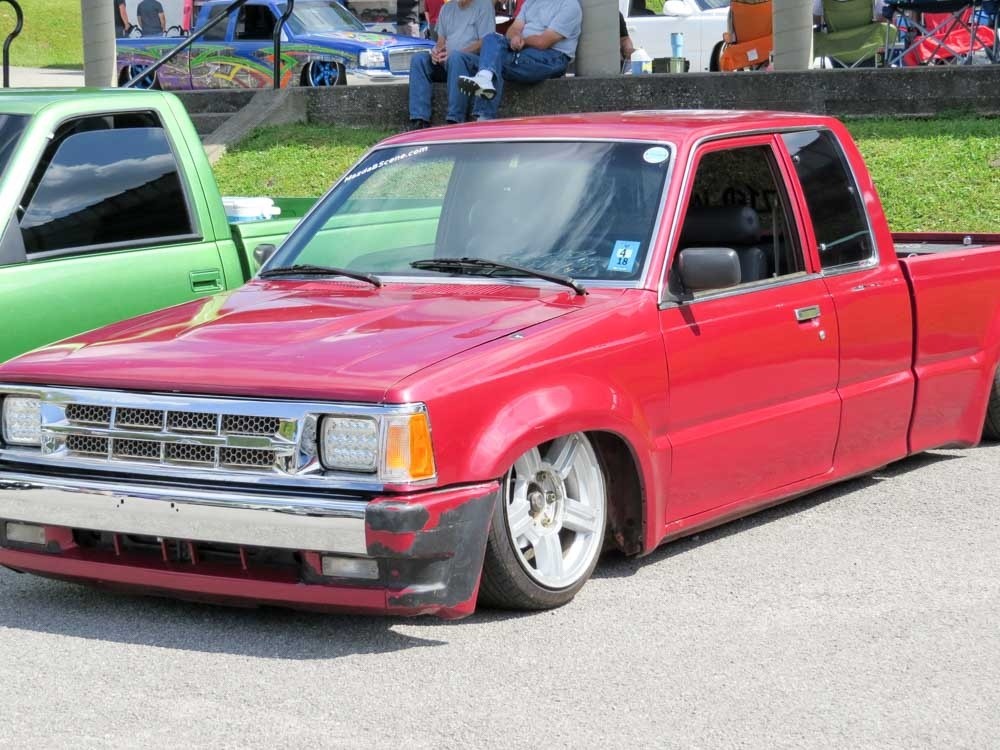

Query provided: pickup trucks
[115,1,437,88]
[0,86,445,363]
[0,109,1000,621]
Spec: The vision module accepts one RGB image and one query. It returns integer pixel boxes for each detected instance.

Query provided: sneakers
[456,75,498,102]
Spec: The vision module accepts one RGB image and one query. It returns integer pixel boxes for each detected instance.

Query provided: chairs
[719,0,774,71]
[813,0,898,69]
[881,0,1000,65]
[625,207,767,290]
[456,196,566,275]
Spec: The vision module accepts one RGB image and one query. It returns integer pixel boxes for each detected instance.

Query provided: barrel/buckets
[223,198,280,222]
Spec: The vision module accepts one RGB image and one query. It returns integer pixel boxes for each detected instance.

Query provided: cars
[618,0,731,73]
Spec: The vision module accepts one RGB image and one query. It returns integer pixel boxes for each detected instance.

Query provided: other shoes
[408,120,431,131]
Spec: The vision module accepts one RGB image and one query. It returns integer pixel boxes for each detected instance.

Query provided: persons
[114,0,201,37]
[813,0,1000,64]
[396,0,582,131]
[620,0,772,73]
[351,7,391,23]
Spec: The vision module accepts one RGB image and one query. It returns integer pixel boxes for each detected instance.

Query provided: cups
[671,32,683,56]
